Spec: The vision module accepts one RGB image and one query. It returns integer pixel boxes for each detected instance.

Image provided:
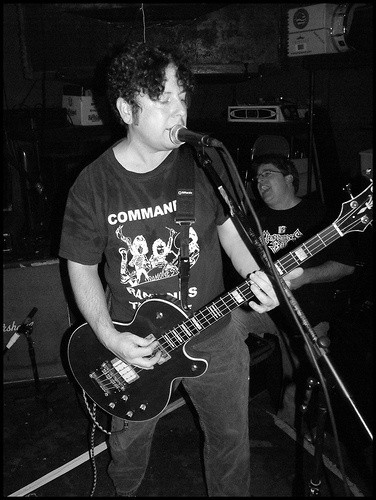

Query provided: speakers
[2,258,76,385]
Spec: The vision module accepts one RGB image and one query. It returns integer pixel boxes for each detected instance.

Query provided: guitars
[66,169,376,423]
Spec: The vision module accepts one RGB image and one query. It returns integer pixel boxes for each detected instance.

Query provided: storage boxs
[278,3,340,60]
[61,84,103,126]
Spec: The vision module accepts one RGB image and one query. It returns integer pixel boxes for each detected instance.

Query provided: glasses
[252,170,284,183]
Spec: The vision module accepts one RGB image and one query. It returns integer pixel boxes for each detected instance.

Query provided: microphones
[169,124,222,147]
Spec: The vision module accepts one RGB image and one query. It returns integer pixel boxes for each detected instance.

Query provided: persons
[247,153,357,336]
[60,42,305,500]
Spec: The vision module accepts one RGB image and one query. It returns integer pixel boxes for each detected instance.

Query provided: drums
[62,85,113,126]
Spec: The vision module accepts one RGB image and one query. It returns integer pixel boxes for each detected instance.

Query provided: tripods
[4,307,57,415]
